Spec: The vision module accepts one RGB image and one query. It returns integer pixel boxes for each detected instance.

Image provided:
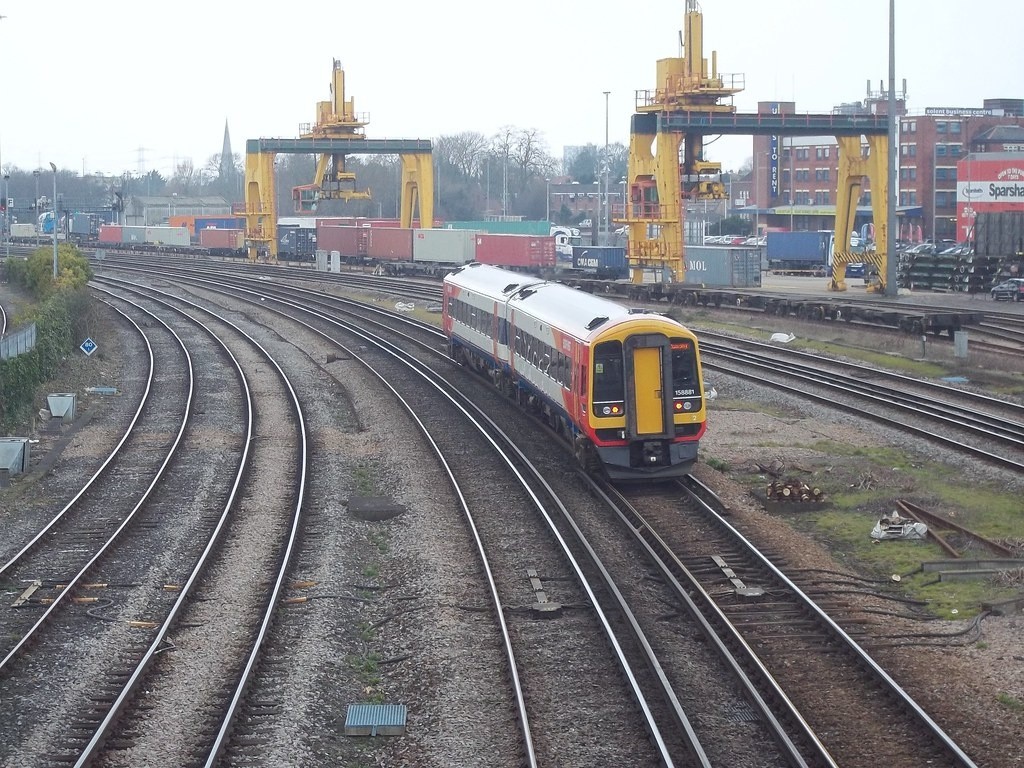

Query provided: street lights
[3,175,10,259]
[618,179,626,235]
[602,91,612,246]
[33,170,40,247]
[571,182,580,210]
[48,162,57,278]
[545,177,552,221]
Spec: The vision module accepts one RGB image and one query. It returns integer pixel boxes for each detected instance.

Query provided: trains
[441,259,709,487]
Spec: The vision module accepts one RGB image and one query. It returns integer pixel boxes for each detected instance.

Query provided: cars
[991,277,1024,302]
[702,228,879,277]
[895,237,974,256]
[578,218,594,228]
[616,225,630,236]
[549,221,582,238]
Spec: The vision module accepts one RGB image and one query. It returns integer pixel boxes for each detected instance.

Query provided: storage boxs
[99,216,836,289]
